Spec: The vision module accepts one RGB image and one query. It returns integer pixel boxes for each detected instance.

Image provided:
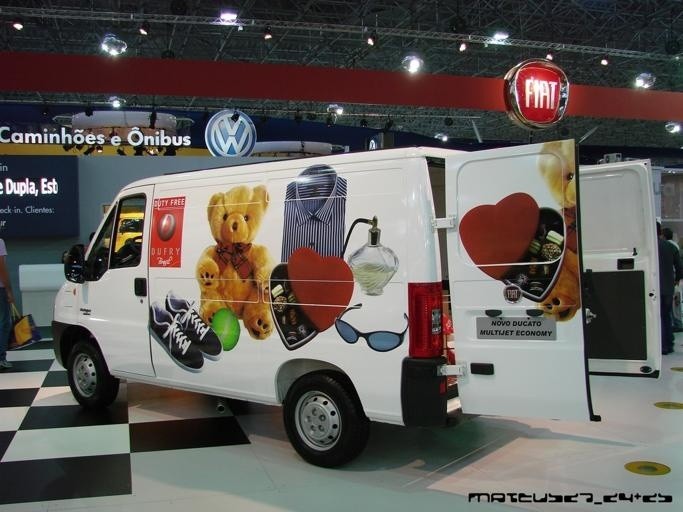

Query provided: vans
[50,138,660,468]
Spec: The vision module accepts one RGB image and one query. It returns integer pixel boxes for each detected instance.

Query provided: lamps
[0,0,273,155]
[294,0,682,162]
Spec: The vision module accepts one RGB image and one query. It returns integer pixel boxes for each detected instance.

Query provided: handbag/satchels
[7,303,41,351]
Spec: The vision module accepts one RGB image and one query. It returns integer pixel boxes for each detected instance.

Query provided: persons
[81,231,95,251]
[124,238,133,244]
[663,228,680,250]
[657,222,682,354]
[0,238,18,369]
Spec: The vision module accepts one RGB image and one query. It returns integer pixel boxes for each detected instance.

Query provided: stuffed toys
[197,184,274,339]
[535,138,582,320]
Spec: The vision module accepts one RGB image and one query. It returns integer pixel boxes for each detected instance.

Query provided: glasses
[335,304,410,352]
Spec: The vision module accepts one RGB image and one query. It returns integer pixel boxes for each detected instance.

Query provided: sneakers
[165,289,224,361]
[146,301,205,373]
[1,359,13,368]
